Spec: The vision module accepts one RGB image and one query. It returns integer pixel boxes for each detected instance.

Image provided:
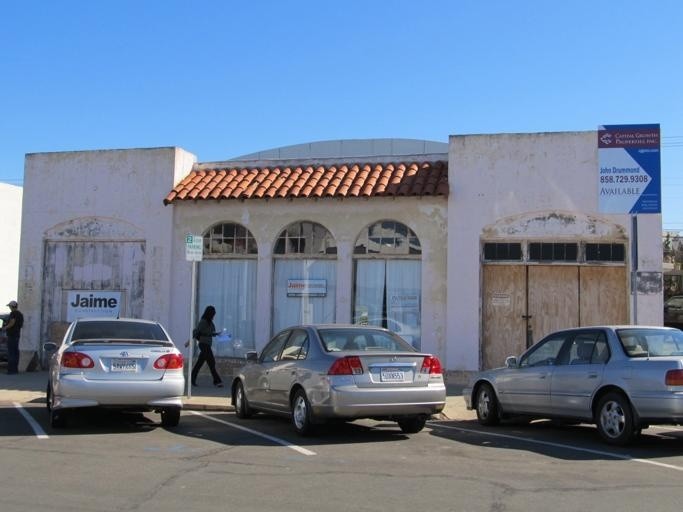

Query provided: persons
[1,299,25,376]
[191,305,223,387]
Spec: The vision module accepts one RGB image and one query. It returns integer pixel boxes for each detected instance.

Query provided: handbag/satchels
[193,328,201,340]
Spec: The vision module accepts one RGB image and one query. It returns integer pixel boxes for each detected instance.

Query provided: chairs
[568,342,603,366]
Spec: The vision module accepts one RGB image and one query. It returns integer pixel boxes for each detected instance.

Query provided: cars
[365,317,420,346]
[41,313,188,430]
[462,323,683,452]
[663,295,683,327]
[228,323,449,440]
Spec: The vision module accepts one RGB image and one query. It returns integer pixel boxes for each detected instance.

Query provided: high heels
[214,383,223,388]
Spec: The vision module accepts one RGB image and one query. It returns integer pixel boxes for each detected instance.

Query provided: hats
[6,301,17,307]
